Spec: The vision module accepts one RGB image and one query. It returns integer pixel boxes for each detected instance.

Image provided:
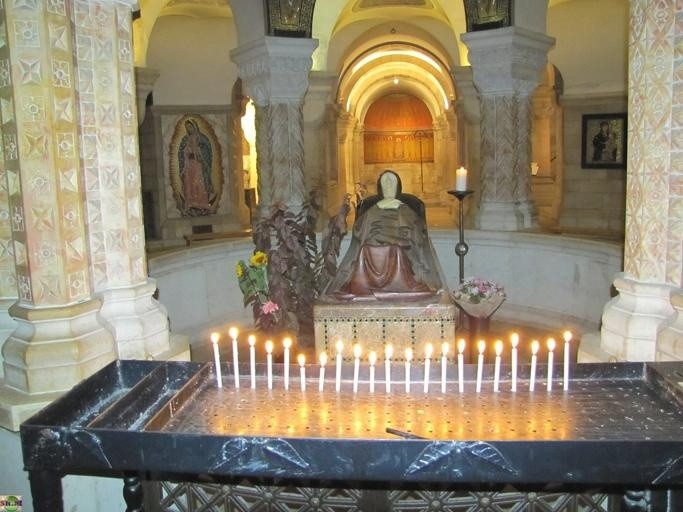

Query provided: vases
[467,318,491,366]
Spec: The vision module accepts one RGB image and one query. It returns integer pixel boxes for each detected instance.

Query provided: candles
[455,166,468,192]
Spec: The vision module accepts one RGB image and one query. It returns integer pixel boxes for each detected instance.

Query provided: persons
[177,119,214,207]
[315,170,443,301]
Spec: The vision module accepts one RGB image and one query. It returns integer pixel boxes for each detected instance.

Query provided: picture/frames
[581,114,627,168]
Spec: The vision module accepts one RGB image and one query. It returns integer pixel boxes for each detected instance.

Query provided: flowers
[235,253,279,327]
[452,276,507,319]
